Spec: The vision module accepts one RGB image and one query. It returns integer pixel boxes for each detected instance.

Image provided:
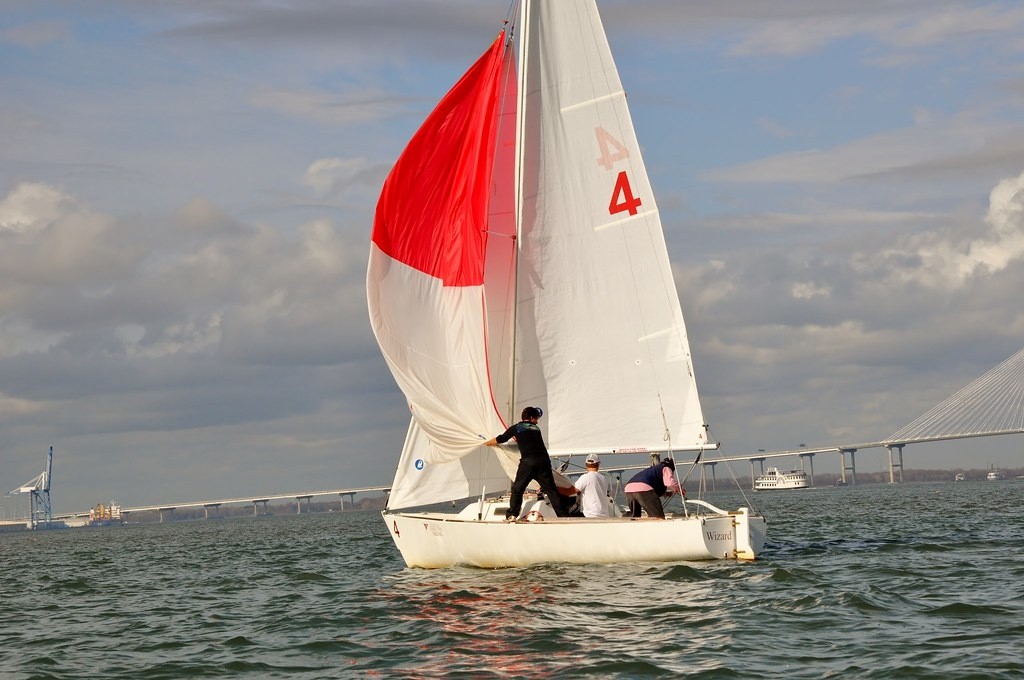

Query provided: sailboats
[366,1,770,565]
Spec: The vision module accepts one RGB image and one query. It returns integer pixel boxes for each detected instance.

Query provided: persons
[96,504,101,518]
[538,453,609,519]
[484,406,569,519]
[625,457,687,520]
[90,507,94,522]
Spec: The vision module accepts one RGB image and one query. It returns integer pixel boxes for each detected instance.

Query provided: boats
[754,464,811,490]
[954,472,965,481]
[987,471,1001,480]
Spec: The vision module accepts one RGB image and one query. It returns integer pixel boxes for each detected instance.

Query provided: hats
[585,454,599,464]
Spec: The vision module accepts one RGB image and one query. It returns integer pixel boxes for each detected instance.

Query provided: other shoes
[508,515,516,522]
